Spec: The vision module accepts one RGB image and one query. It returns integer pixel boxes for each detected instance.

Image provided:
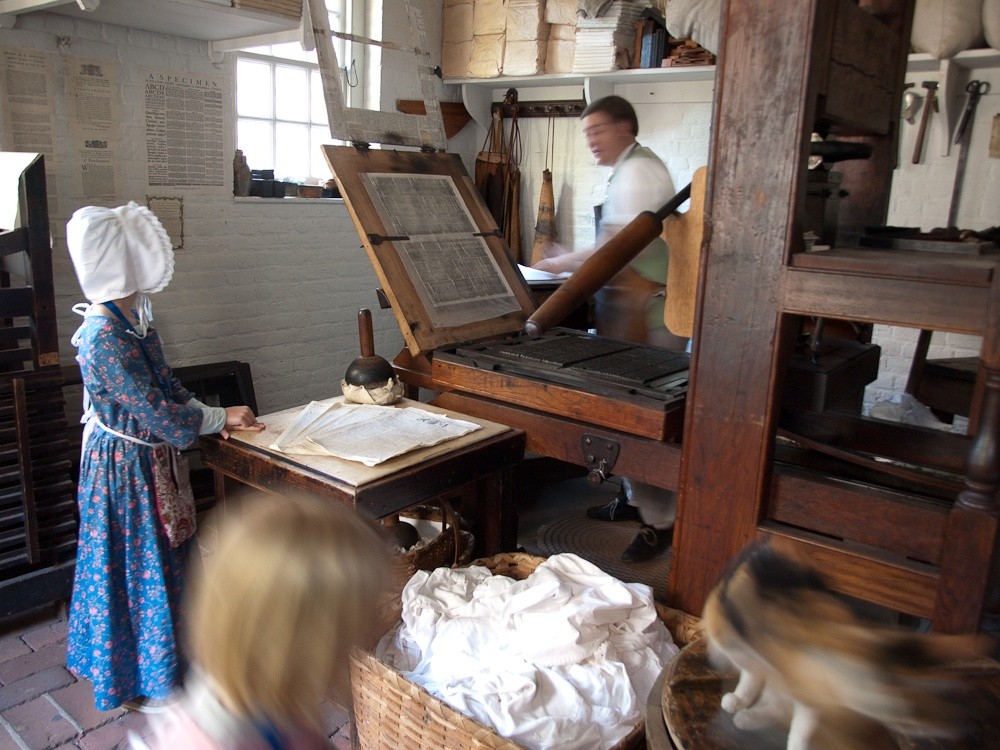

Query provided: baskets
[347,552,709,750]
[386,497,460,578]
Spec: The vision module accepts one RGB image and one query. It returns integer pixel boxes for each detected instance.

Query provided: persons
[66,199,266,712]
[528,96,688,566]
[119,494,404,750]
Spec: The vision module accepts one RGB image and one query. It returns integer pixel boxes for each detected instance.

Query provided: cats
[693,528,1000,750]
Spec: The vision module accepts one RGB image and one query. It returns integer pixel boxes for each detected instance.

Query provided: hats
[67,202,175,304]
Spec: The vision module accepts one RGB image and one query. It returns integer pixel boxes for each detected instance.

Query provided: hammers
[912,81,939,164]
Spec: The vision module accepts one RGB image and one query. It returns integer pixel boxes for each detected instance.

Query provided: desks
[201,393,526,648]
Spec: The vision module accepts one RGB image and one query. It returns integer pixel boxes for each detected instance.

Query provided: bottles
[233,149,251,196]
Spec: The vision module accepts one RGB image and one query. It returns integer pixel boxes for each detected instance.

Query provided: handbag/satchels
[151,441,196,546]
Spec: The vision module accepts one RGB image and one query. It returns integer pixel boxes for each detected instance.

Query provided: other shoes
[621,522,672,562]
[121,688,185,712]
[587,501,640,522]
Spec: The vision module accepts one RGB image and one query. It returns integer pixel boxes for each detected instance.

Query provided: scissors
[954,80,990,144]
